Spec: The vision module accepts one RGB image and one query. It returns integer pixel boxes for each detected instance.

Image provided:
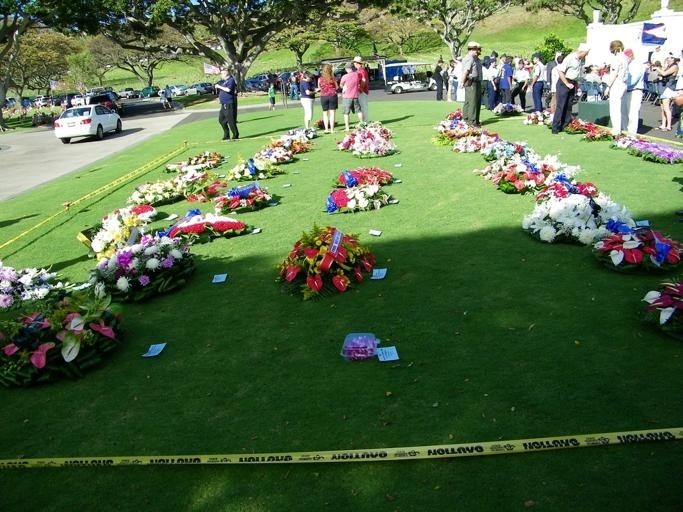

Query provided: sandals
[655,126,667,131]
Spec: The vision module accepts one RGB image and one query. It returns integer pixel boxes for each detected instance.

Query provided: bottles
[594,94,597,103]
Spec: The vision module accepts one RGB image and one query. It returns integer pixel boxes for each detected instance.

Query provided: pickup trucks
[118,88,140,99]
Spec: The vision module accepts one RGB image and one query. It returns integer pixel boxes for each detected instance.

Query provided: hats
[579,43,591,52]
[352,56,363,63]
[468,42,482,49]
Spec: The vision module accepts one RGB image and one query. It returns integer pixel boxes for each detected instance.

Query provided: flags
[203,63,219,75]
[49,80,59,91]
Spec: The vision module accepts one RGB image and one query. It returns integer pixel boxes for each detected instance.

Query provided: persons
[298,71,317,139]
[315,65,339,134]
[264,63,375,110]
[213,68,239,142]
[429,38,682,141]
[349,56,369,127]
[159,91,171,110]
[338,62,363,132]
[164,85,172,107]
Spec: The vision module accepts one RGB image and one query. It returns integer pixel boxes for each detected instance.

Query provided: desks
[575,98,611,126]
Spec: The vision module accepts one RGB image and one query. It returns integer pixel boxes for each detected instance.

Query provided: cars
[333,62,369,76]
[138,85,159,97]
[183,82,212,96]
[53,105,122,143]
[157,84,186,96]
[210,80,223,94]
[5,84,116,107]
[241,62,296,92]
